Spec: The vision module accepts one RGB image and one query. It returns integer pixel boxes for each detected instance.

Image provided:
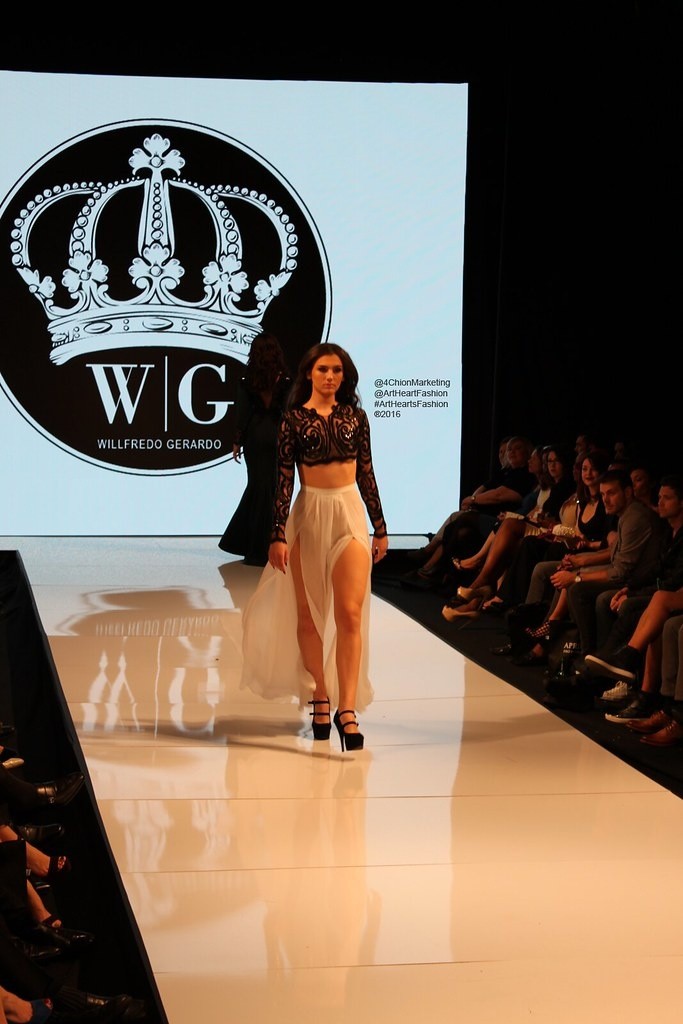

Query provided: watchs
[575,572,582,584]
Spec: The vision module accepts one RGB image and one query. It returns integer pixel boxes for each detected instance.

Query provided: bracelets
[471,495,476,502]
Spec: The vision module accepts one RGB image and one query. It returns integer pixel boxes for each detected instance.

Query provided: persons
[268,342,390,753]
[0,665,135,1024]
[410,428,683,748]
[231,296,305,566]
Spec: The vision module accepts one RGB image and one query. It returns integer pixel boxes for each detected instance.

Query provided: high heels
[406,546,597,698]
[333,709,364,753]
[307,697,331,740]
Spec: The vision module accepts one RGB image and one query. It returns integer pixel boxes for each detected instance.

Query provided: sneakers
[604,696,658,724]
[583,647,639,681]
[600,682,638,701]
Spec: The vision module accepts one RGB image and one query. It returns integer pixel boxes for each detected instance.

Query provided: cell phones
[564,539,577,550]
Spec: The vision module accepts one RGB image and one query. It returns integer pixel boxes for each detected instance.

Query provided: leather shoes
[640,717,683,748]
[0,724,147,1024]
[627,709,670,733]
